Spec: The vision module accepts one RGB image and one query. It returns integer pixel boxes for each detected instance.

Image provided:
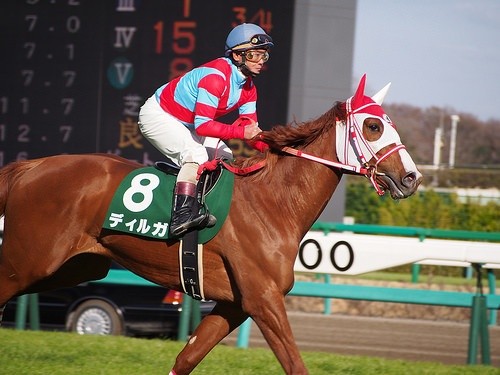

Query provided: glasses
[237,50,270,62]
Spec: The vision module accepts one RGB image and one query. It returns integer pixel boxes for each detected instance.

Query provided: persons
[139,23,274,237]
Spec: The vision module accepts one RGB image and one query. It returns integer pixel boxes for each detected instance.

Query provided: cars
[2,260,219,336]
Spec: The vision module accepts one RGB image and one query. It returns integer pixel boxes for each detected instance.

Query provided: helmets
[225,23,274,55]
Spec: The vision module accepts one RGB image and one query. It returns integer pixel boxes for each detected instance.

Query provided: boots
[170,182,217,235]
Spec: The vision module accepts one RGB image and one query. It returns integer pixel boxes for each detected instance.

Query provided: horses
[0,73,423,375]
